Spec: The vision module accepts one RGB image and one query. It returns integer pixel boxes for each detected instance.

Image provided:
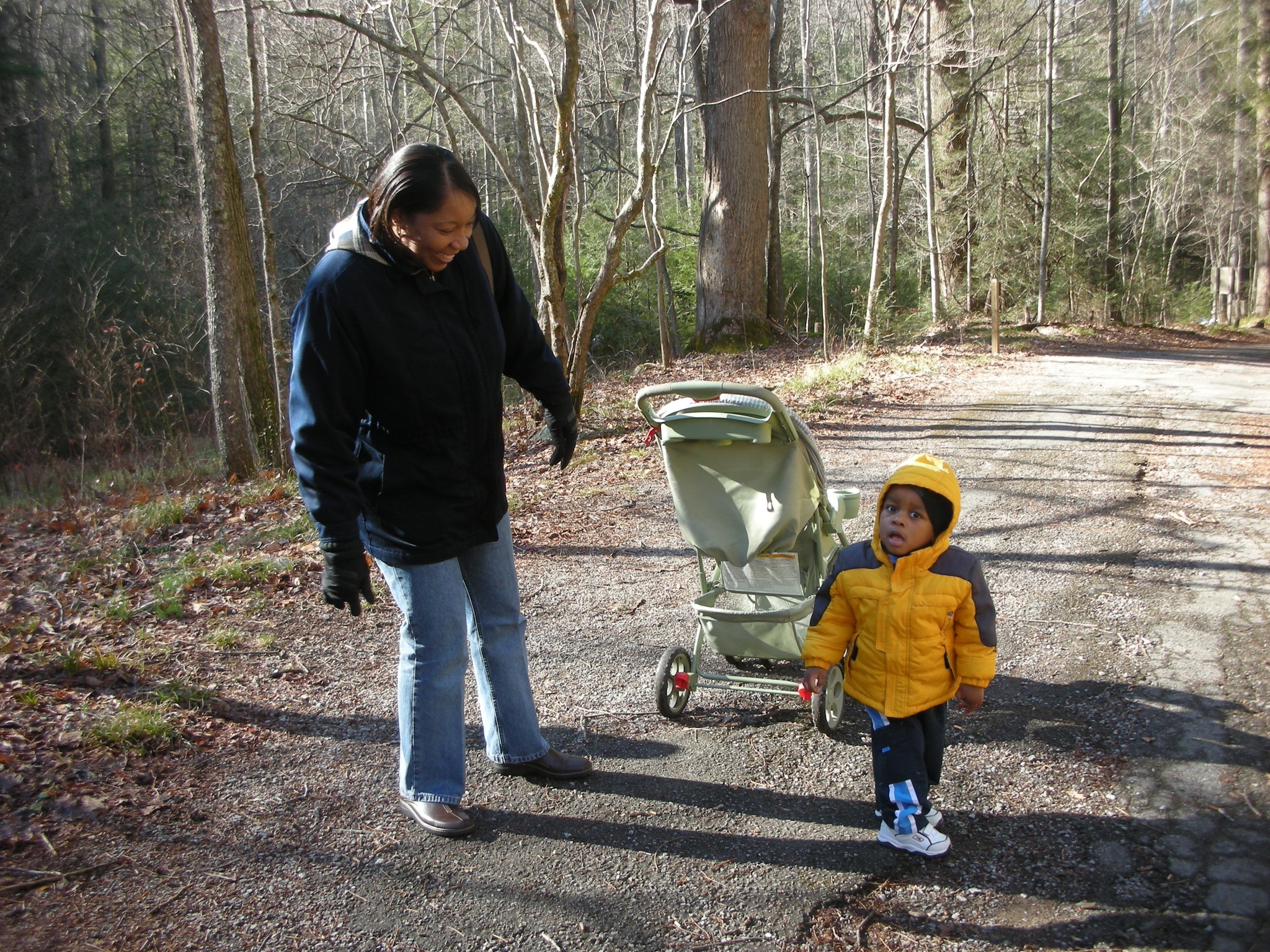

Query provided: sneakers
[876,819,950,859]
[875,803,944,828]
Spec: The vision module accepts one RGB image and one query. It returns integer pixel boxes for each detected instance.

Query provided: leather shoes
[399,793,475,836]
[494,745,592,779]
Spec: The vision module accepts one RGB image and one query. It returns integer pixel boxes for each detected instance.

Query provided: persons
[290,144,593,839]
[800,454,996,859]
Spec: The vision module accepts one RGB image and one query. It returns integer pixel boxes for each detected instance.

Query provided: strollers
[634,381,867,733]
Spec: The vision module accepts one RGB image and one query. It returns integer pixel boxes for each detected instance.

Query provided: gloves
[319,539,374,616]
[544,392,577,469]
